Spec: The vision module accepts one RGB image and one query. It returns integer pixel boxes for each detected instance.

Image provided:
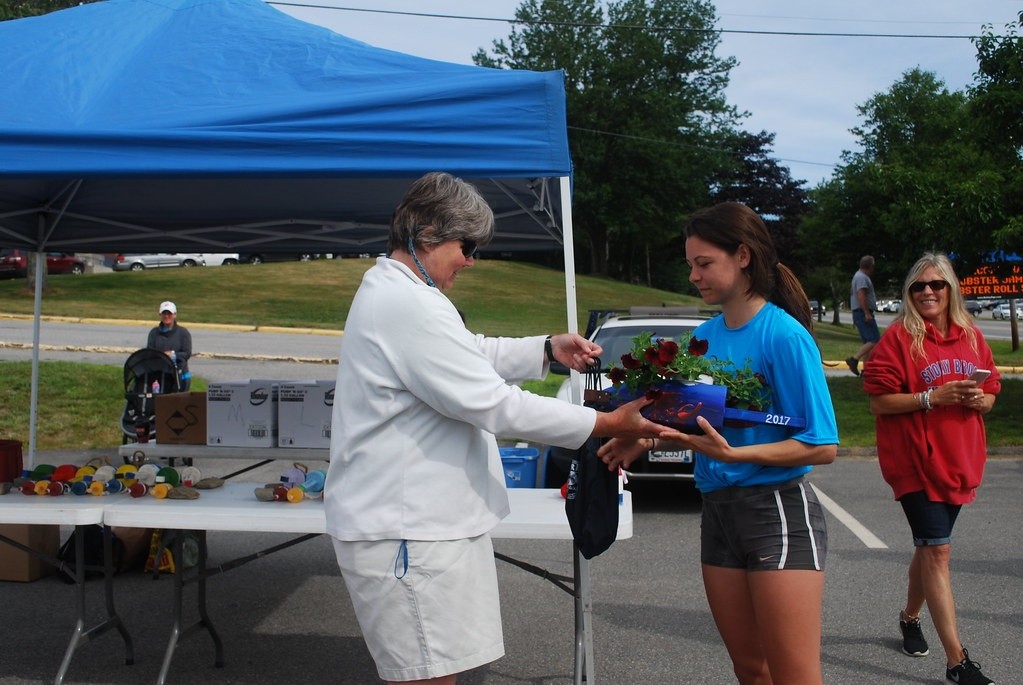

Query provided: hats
[159,301,177,313]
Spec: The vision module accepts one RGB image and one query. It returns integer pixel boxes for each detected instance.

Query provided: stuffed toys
[19,464,201,498]
[273,486,304,504]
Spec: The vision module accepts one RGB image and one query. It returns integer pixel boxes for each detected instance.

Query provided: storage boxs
[207,380,278,447]
[500,443,540,488]
[277,380,336,450]
[1,525,60,582]
[154,391,209,444]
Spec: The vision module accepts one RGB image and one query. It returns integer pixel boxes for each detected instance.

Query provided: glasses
[460,236,479,259]
[909,279,951,292]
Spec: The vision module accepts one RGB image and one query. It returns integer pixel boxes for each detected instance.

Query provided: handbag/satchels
[49,525,126,584]
[565,356,621,559]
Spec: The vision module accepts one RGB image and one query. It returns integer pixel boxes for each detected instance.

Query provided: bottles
[171,351,176,366]
[618,465,623,505]
[153,381,160,394]
[135,411,150,444]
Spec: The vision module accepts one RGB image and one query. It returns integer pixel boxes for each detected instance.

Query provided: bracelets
[649,437,655,449]
[545,335,560,364]
[912,389,935,410]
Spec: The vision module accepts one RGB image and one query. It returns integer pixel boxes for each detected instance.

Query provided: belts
[853,308,873,312]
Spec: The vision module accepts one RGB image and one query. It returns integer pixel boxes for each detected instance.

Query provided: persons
[147,301,193,392]
[324,171,685,685]
[862,251,1000,685]
[596,203,840,685]
[844,256,879,376]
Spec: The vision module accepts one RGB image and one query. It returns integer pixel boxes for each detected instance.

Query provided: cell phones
[967,369,991,389]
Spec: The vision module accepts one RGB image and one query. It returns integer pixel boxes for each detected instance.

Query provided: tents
[0,0,602,685]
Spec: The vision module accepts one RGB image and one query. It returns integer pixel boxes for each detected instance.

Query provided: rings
[974,395,977,399]
[961,395,965,399]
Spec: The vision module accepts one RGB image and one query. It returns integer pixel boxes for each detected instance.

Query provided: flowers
[606,332,768,415]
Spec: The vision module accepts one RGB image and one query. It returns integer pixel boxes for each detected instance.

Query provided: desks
[0,483,634,685]
[119,439,332,461]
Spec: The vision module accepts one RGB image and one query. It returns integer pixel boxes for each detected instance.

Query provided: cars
[0,249,27,277]
[980,300,1009,311]
[809,301,826,316]
[47,252,85,276]
[963,301,982,317]
[1013,299,1023,306]
[549,302,725,478]
[112,253,206,271]
[992,304,1011,321]
[1015,303,1023,319]
[876,300,902,312]
[202,253,239,266]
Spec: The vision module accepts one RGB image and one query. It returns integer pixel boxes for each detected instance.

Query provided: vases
[604,379,728,429]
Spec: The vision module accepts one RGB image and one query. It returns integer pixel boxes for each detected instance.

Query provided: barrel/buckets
[498,448,540,489]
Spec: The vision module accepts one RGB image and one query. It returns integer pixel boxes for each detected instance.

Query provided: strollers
[119,348,180,464]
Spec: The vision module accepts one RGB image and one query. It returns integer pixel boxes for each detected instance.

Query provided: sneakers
[845,356,860,375]
[899,610,929,655]
[946,643,996,685]
